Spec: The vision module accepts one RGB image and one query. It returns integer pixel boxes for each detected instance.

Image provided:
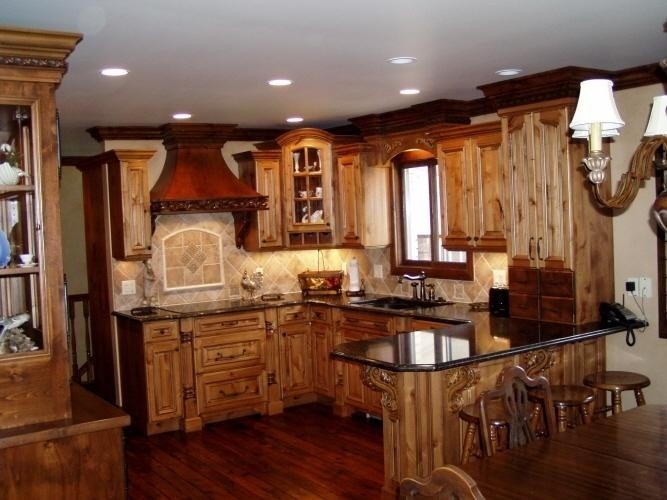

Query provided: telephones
[598,300,638,346]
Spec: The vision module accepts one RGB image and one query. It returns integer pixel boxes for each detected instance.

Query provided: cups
[19,254,32,264]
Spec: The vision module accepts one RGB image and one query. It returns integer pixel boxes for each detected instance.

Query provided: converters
[626,281,636,292]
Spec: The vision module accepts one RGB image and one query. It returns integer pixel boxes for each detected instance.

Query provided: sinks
[352,295,445,311]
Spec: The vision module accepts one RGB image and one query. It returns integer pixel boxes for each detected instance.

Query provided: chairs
[379,464,484,500]
[476,364,557,458]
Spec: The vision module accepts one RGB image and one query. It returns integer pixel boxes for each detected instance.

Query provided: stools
[551,385,596,434]
[458,401,541,465]
[582,371,651,416]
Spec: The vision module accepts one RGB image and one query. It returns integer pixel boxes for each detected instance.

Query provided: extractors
[150,131,267,214]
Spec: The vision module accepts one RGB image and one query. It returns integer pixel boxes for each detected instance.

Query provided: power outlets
[625,277,639,297]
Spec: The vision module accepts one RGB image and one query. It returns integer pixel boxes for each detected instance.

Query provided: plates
[17,264,39,268]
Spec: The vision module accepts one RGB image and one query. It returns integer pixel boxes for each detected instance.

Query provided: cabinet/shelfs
[109,158,152,262]
[277,304,335,411]
[141,317,185,440]
[0,65,72,433]
[332,308,393,422]
[249,137,365,250]
[497,97,616,326]
[181,308,282,436]
[437,130,508,253]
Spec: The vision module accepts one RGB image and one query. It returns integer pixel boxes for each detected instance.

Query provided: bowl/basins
[298,191,312,198]
[315,186,322,197]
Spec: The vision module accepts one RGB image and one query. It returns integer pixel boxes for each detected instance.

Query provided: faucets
[397,272,435,302]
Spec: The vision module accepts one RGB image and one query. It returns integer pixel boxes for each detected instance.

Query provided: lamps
[567,77,667,217]
